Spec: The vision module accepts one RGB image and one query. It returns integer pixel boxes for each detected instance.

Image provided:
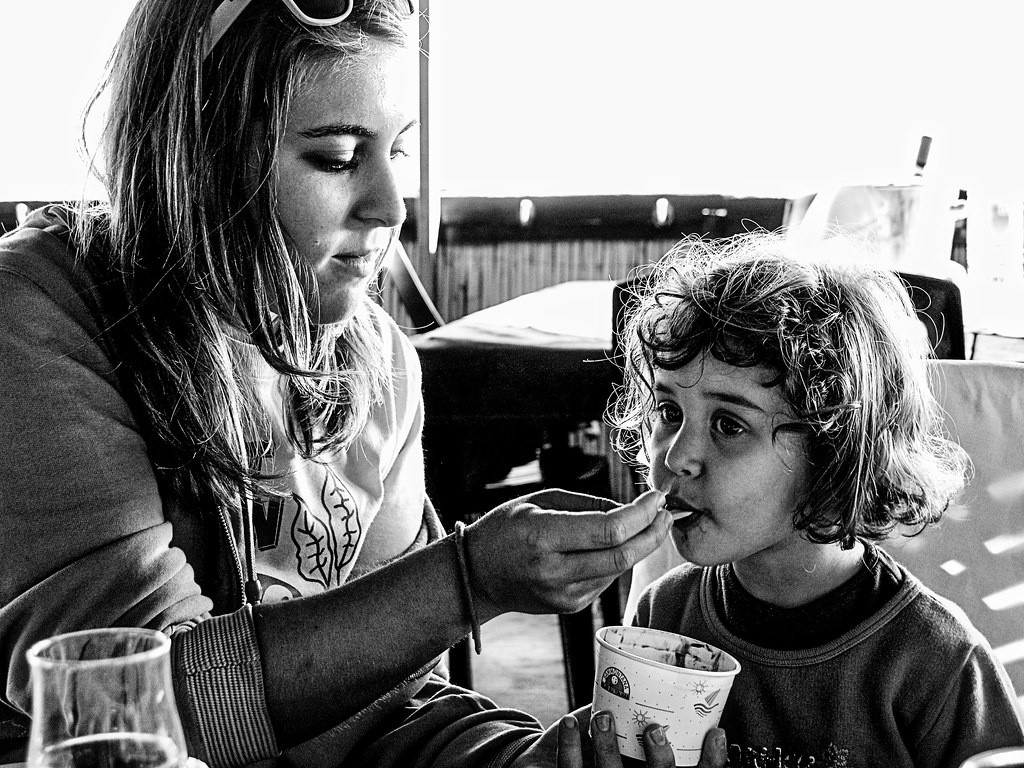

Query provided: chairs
[878,360,1024,719]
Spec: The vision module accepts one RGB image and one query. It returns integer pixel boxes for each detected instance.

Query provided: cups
[26,627,188,768]
[588,626,741,767]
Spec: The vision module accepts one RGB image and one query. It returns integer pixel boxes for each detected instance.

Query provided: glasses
[201,0,414,63]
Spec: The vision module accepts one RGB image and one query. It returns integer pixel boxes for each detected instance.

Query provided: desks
[410,280,632,713]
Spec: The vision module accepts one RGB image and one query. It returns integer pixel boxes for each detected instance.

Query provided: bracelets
[453,521,482,655]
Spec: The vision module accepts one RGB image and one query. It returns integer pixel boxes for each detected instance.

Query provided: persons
[623,234,1023,768]
[0,0,730,768]
